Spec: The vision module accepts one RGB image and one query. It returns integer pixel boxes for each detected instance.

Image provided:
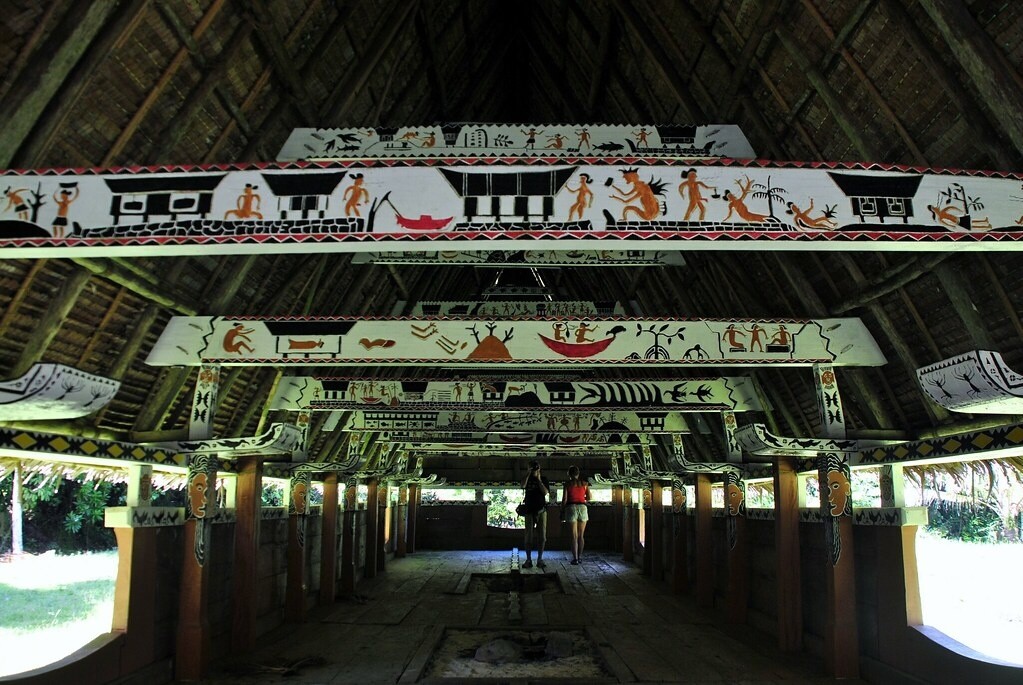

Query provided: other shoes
[522,560,533,568]
[536,559,545,568]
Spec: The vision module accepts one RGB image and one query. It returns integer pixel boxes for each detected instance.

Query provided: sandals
[578,558,582,563]
[572,559,579,565]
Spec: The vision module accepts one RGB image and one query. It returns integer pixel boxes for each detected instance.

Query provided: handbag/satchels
[516,504,528,516]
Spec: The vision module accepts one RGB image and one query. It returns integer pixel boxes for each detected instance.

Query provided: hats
[530,462,540,470]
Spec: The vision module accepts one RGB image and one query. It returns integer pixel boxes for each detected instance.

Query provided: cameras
[530,466,539,471]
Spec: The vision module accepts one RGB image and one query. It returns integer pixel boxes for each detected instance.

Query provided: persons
[521,460,549,569]
[561,465,592,565]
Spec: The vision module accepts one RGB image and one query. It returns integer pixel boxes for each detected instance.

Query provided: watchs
[540,482,544,486]
